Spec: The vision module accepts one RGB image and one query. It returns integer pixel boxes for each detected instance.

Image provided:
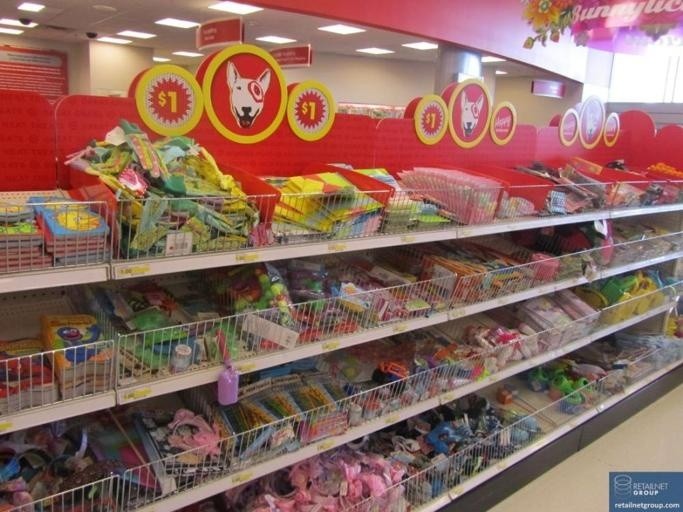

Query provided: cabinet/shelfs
[0,180,682,512]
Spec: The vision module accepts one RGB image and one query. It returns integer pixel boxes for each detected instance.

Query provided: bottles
[172,344,191,372]
